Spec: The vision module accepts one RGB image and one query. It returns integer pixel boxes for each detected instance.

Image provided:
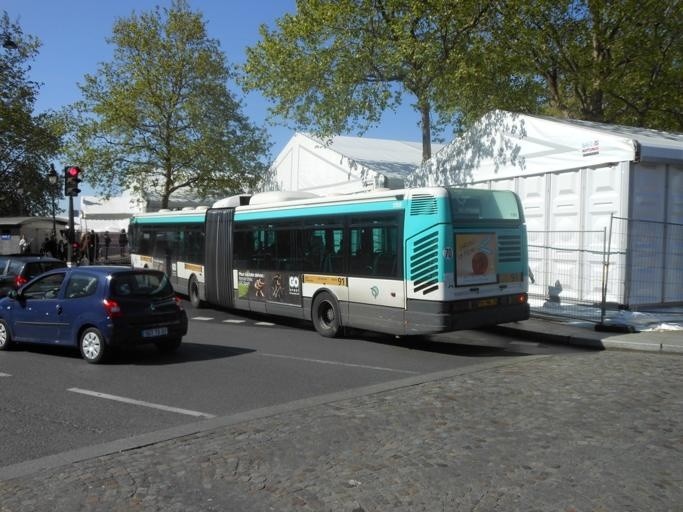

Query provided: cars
[0,256,188,364]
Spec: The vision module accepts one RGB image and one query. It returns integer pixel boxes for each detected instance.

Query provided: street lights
[47,164,59,234]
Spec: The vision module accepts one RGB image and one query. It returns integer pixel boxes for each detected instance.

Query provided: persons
[119,228,128,257]
[17,233,26,255]
[254,278,265,297]
[103,230,113,259]
[21,237,32,255]
[39,228,99,265]
[271,273,281,297]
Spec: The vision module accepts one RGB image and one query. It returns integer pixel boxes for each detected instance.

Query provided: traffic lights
[65,167,82,196]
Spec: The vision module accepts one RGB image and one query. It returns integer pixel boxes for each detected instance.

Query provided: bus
[129,187,530,338]
